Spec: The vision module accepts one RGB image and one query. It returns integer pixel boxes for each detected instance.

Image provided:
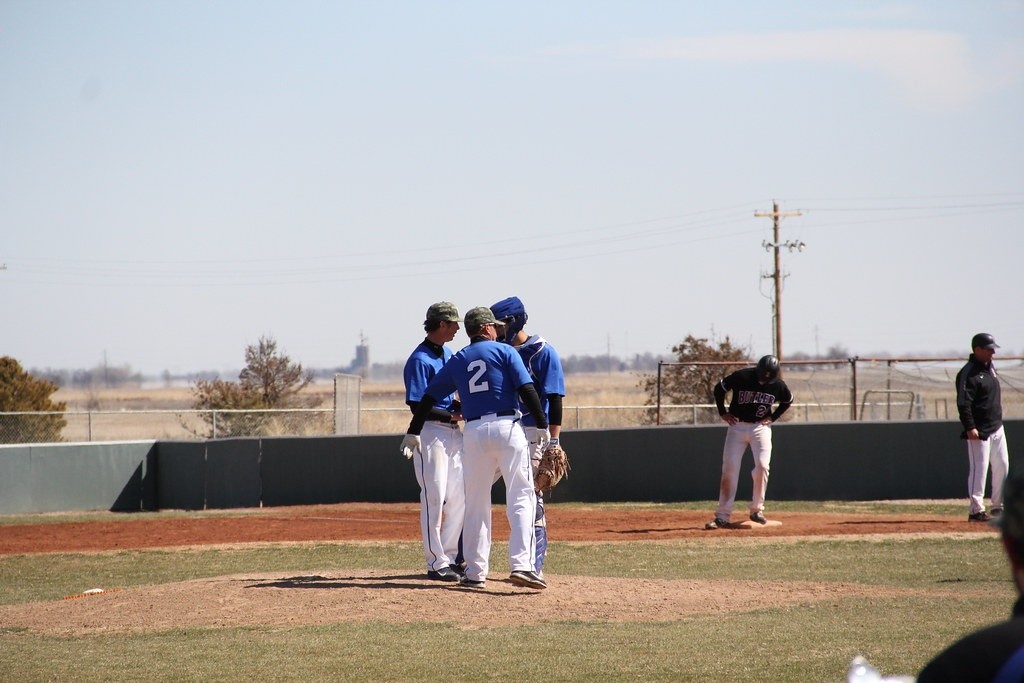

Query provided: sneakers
[457,578,484,588]
[449,563,465,578]
[510,571,547,589]
[428,567,460,582]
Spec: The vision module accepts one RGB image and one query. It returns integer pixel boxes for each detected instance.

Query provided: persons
[914,452,1024,683]
[705,355,793,530]
[486,296,565,579]
[402,300,467,580]
[399,305,551,588]
[955,333,1010,521]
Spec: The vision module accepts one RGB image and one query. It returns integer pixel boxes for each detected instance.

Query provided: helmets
[972,333,1001,349]
[756,355,781,384]
[988,465,1024,535]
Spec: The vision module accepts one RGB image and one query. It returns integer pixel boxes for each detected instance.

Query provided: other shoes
[749,512,766,524]
[458,561,467,570]
[968,512,994,522]
[990,510,1003,516]
[705,518,728,529]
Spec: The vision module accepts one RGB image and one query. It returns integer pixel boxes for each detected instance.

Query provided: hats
[464,306,506,328]
[489,296,524,319]
[427,301,463,322]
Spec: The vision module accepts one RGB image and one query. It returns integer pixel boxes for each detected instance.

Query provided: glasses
[482,322,498,329]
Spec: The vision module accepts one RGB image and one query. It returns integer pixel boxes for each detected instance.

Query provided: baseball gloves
[534,445,568,491]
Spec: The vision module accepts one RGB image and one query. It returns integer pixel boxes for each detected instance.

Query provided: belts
[450,420,457,424]
[467,411,515,423]
[737,417,762,423]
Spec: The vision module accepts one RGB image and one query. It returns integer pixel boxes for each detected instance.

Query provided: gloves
[960,431,990,441]
[536,426,551,446]
[400,434,422,459]
